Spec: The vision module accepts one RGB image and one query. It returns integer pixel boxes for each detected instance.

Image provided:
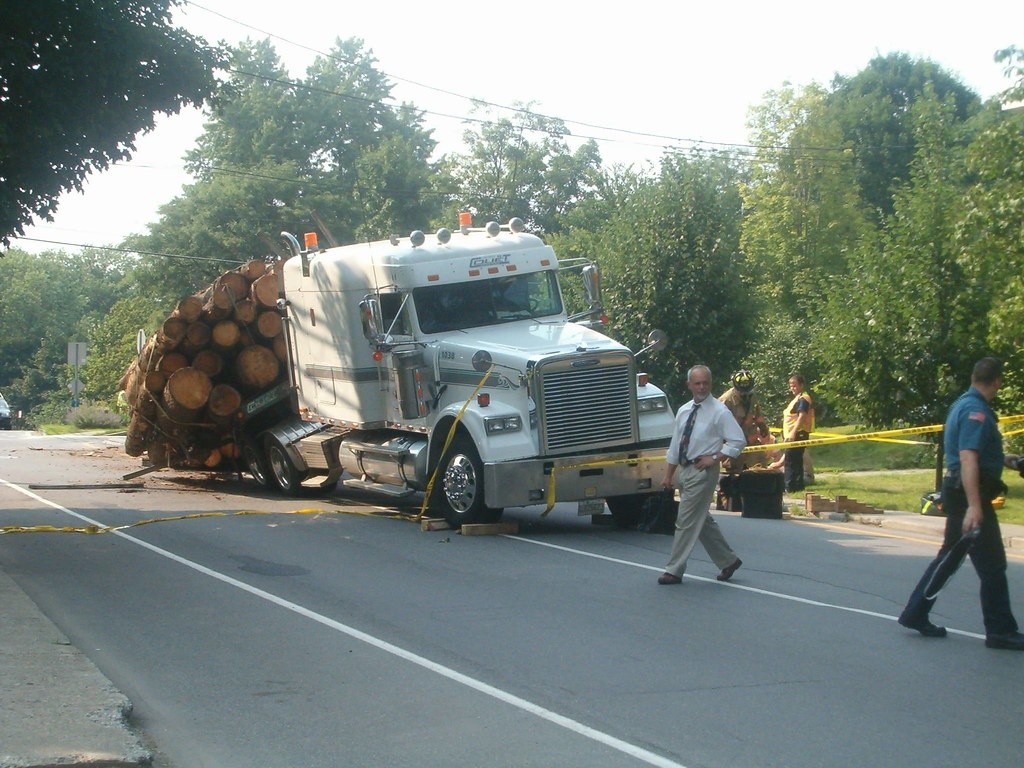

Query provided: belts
[687,460,698,464]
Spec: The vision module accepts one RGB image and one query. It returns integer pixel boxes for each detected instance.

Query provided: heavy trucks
[239,212,677,532]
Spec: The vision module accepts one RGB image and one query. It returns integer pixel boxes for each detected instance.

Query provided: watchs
[712,454,719,462]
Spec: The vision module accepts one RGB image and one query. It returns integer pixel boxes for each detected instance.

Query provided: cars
[0,396,12,430]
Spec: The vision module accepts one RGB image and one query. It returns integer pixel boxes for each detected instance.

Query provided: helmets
[732,369,760,393]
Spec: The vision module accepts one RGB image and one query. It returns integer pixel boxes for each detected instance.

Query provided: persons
[898,359,1024,648]
[119,388,128,414]
[658,365,747,585]
[718,371,768,473]
[783,376,815,493]
[756,425,814,485]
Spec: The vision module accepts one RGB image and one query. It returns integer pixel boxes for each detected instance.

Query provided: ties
[678,404,702,467]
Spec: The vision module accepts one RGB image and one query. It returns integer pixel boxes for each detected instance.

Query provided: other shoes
[897,609,947,638]
[657,572,682,584]
[716,557,742,581]
[985,630,1024,650]
